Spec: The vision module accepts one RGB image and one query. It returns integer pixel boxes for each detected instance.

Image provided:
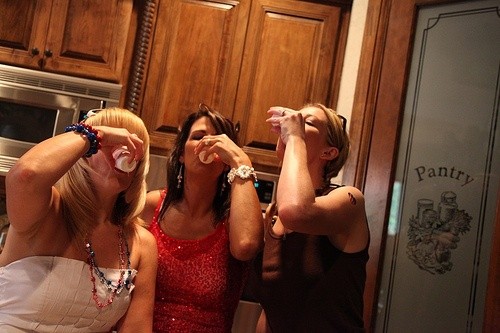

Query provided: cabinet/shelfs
[1,0,135,84]
[125,0,348,176]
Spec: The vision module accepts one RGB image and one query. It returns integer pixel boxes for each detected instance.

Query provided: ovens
[0,63,124,177]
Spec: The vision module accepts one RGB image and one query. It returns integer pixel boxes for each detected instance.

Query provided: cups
[112,145,137,173]
[270,113,281,127]
[198,146,215,164]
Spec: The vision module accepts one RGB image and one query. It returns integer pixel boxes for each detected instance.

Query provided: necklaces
[85,219,133,290]
[81,222,126,308]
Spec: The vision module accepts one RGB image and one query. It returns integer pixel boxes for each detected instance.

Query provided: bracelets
[227,162,259,186]
[64,124,98,158]
[73,122,103,155]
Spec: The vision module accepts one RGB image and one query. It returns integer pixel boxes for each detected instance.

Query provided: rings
[281,109,286,117]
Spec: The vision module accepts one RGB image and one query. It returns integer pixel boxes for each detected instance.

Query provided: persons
[137,101,265,333]
[232,102,371,333]
[1,107,159,333]
